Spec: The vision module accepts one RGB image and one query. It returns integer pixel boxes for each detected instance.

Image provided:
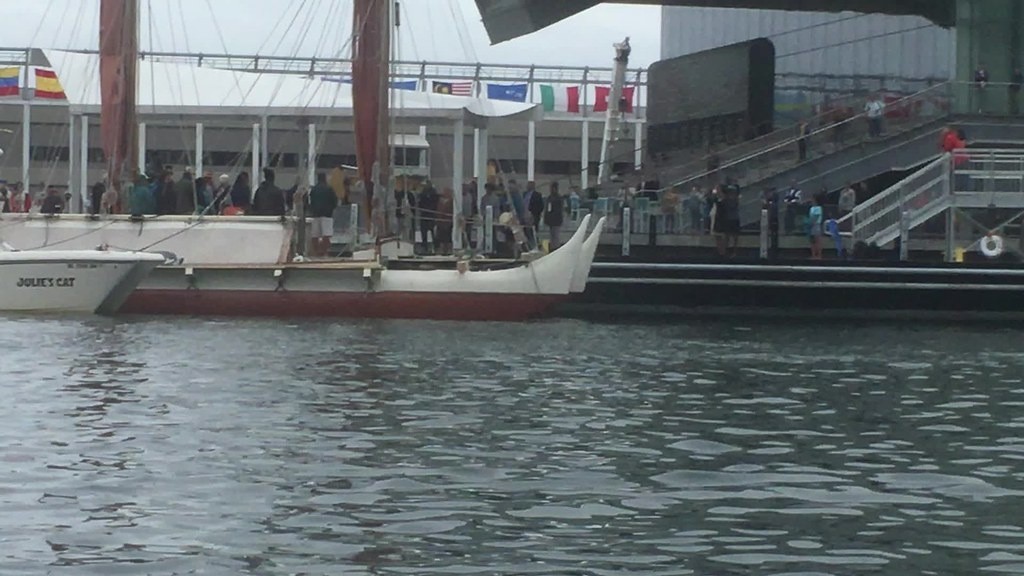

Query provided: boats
[0,0,606,323]
[2,249,168,318]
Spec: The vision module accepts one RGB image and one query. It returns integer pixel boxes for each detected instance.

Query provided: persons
[1007,65,1023,115]
[865,96,883,136]
[0,126,977,262]
[972,61,991,115]
[798,120,809,162]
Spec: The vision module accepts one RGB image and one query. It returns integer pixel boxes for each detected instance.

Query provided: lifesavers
[980,235,1003,257]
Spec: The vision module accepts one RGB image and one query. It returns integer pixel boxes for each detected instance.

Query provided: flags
[540,84,580,112]
[390,81,427,96]
[593,86,635,112]
[431,81,473,97]
[488,84,528,103]
